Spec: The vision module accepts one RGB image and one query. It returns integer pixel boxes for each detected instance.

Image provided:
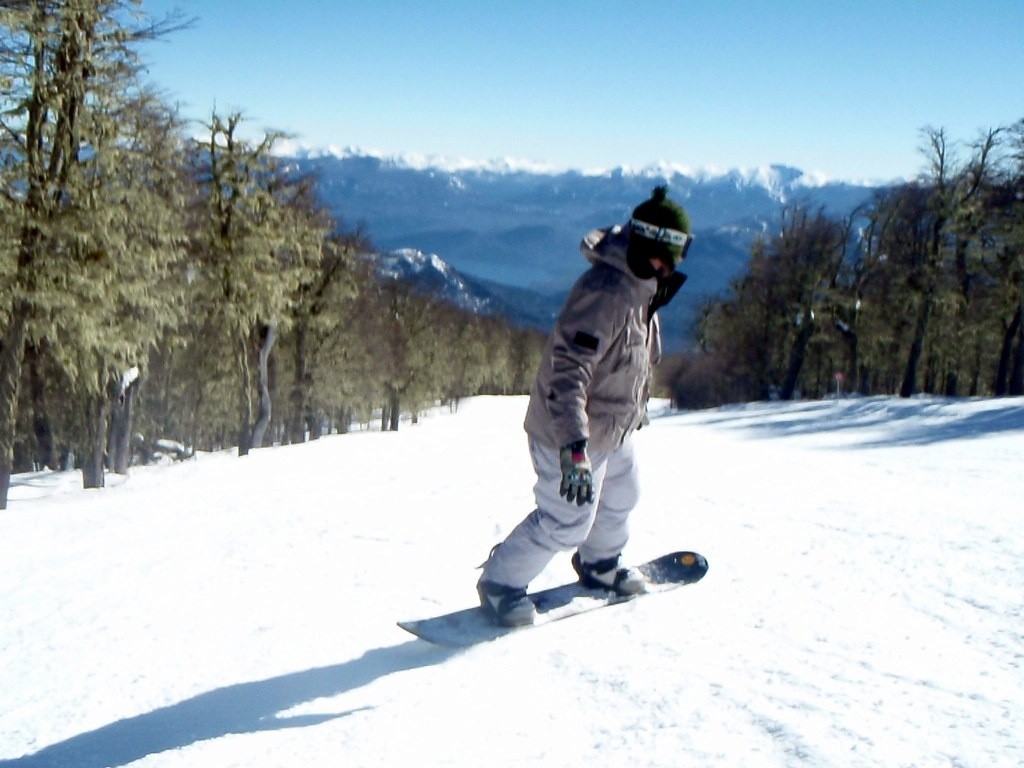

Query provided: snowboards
[396,551,709,649]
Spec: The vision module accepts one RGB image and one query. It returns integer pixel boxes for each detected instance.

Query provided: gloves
[558,438,596,507]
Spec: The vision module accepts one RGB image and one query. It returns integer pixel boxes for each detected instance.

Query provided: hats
[626,185,691,268]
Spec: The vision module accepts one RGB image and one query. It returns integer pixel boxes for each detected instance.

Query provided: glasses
[682,236,694,259]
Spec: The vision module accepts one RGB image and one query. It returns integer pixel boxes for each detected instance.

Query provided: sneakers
[476,575,538,626]
[571,549,645,603]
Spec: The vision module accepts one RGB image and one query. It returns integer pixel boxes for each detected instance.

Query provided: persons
[475,183,692,627]
